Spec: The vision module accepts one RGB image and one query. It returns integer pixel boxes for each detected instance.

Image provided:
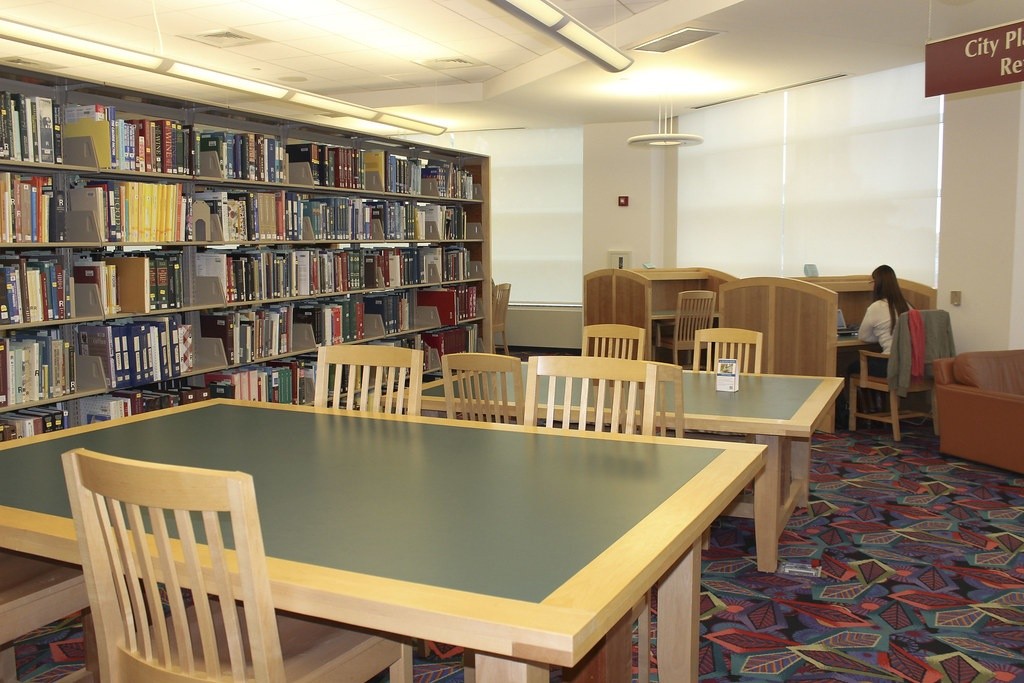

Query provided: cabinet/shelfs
[0,58,494,413]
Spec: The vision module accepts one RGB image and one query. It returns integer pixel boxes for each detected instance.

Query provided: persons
[847,265,917,432]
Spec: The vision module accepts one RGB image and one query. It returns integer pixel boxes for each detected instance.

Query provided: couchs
[934,349,1024,477]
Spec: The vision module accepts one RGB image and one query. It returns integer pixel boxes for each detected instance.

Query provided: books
[0,87,486,443]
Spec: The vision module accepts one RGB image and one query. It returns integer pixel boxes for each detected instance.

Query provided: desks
[583,266,741,362]
[380,358,846,573]
[0,396,769,683]
[718,274,939,435]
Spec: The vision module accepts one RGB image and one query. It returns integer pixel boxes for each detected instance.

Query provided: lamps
[627,98,705,150]
[495,0,634,72]
[0,11,448,137]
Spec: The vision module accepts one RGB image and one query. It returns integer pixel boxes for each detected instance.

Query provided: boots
[857,390,876,428]
[871,389,887,429]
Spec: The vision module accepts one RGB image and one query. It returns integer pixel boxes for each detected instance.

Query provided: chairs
[661,289,717,372]
[849,309,957,442]
[440,352,524,430]
[581,323,647,361]
[523,355,658,441]
[0,445,417,683]
[693,327,764,376]
[593,359,685,439]
[312,344,425,417]
[492,282,512,357]
